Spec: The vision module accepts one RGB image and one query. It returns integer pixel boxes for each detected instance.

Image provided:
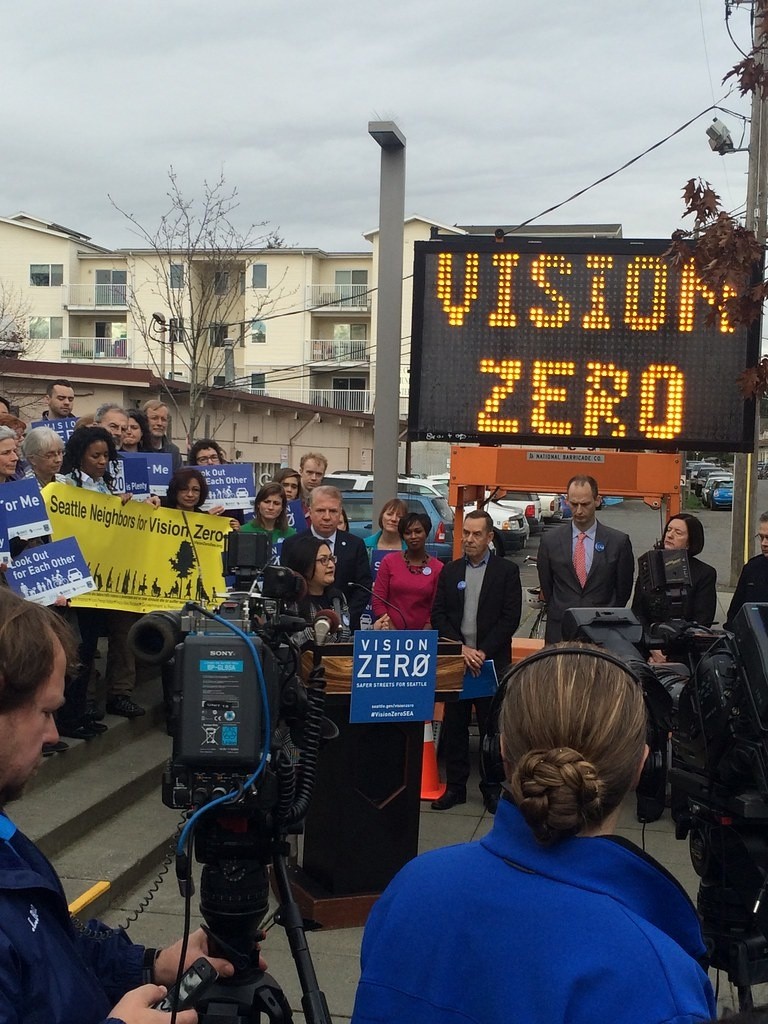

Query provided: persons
[371,513,444,631]
[632,513,716,824]
[363,498,409,566]
[431,510,522,814]
[0,379,182,757]
[190,439,246,526]
[349,642,717,1024]
[723,512,768,634]
[281,485,390,643]
[0,584,269,1024]
[164,467,241,533]
[537,475,635,644]
[239,452,327,544]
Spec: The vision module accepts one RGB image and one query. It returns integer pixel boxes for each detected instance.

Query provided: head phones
[479,646,664,799]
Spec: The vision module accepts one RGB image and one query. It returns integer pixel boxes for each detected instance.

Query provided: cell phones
[150,957,219,1013]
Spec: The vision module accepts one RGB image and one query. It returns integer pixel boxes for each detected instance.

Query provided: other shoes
[107,697,145,717]
[44,745,55,755]
[88,704,105,721]
[483,794,499,814]
[431,790,466,810]
[55,741,69,751]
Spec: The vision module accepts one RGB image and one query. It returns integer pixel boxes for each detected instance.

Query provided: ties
[573,533,587,589]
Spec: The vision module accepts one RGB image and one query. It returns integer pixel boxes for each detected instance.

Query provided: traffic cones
[421,717,447,802]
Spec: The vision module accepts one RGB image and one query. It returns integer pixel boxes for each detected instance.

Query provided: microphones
[315,609,339,634]
[347,582,407,631]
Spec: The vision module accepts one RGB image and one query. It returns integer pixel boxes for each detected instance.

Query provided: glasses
[36,450,67,460]
[755,533,768,541]
[315,556,338,566]
[196,454,219,462]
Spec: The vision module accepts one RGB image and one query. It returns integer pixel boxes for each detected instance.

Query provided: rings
[217,512,220,515]
[470,660,475,663]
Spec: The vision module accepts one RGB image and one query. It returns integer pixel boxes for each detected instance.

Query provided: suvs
[308,469,623,560]
[339,488,456,560]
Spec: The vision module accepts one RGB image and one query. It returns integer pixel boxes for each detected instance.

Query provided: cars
[686,460,733,511]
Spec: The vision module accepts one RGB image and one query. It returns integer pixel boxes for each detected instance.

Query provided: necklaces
[404,548,430,574]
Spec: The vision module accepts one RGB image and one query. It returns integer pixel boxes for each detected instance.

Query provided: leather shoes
[84,720,108,733]
[59,726,96,739]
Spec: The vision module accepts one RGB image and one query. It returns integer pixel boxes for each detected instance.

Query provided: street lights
[153,310,167,378]
[367,118,407,540]
